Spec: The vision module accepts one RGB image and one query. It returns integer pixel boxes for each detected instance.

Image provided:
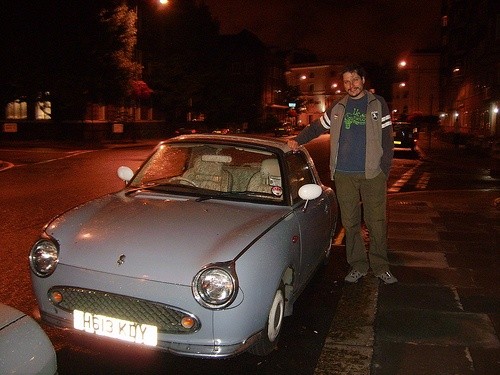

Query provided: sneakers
[344,270,368,283]
[376,271,398,284]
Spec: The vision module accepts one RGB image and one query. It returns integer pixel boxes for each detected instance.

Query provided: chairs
[247,158,283,198]
[179,156,234,192]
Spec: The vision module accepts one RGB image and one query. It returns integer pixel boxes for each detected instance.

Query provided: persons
[286,63,399,285]
[453,119,461,131]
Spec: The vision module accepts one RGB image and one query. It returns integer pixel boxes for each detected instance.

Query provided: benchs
[228,163,262,193]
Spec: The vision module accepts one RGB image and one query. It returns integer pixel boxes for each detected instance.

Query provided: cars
[391,130,414,155]
[28,131,339,361]
[0,301,60,375]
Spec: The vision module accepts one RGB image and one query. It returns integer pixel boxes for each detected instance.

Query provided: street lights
[401,58,421,113]
[327,83,341,109]
[400,82,415,114]
[293,74,306,127]
